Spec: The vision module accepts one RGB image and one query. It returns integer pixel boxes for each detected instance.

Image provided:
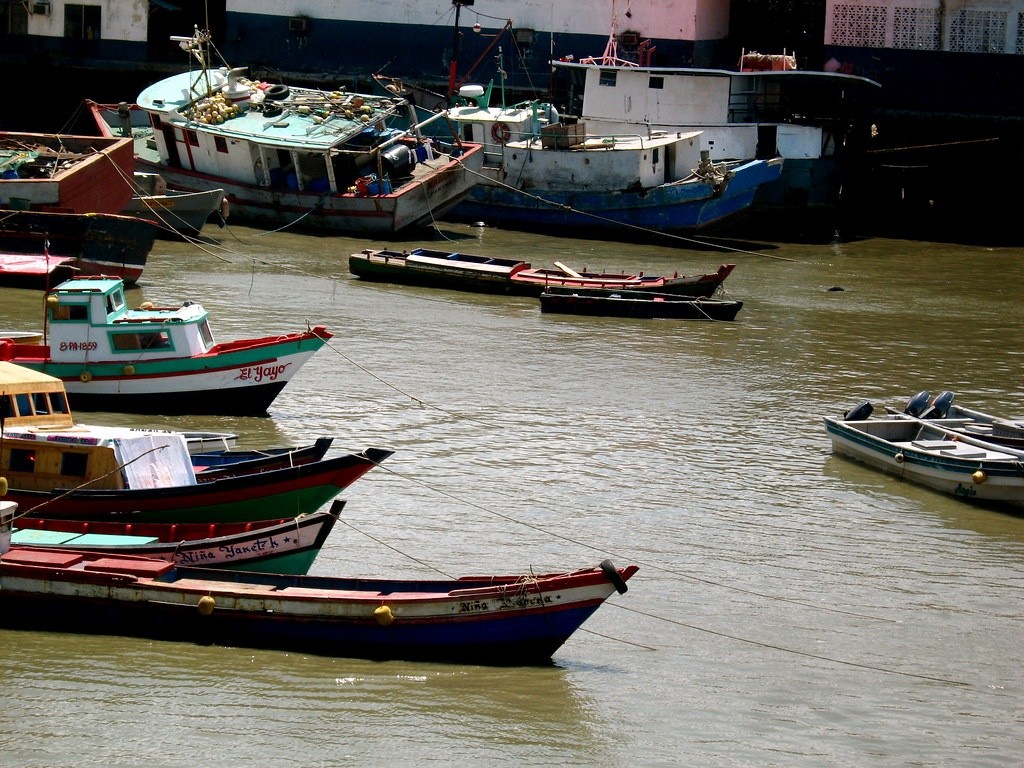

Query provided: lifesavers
[491,123,511,143]
[261,106,281,118]
[265,84,289,101]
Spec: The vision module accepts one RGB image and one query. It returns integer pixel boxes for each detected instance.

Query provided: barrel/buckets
[367,180,390,194]
[374,130,391,144]
[355,176,371,195]
[271,167,283,185]
[372,144,415,178]
[287,175,297,192]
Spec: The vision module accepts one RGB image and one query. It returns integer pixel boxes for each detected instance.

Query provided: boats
[822,405,1024,501]
[349,246,737,298]
[0,501,641,666]
[540,284,743,322]
[883,394,1024,454]
[0,1,786,576]
